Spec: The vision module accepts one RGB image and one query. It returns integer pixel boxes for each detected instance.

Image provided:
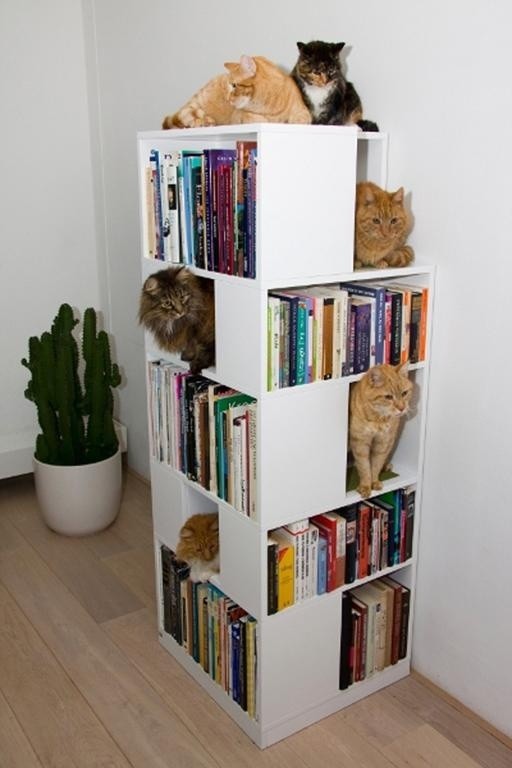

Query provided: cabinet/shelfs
[135,121,434,751]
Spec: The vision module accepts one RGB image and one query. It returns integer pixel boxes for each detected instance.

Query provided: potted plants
[20,303,124,539]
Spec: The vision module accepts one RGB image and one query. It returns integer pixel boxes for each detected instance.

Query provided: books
[147,141,256,279]
[267,482,412,615]
[161,544,257,720]
[148,359,258,518]
[267,282,427,392]
[339,576,411,690]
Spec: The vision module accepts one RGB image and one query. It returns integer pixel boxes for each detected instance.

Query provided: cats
[162,55,312,130]
[174,512,220,584]
[354,181,415,269]
[135,264,216,377]
[348,359,418,498]
[289,40,379,132]
